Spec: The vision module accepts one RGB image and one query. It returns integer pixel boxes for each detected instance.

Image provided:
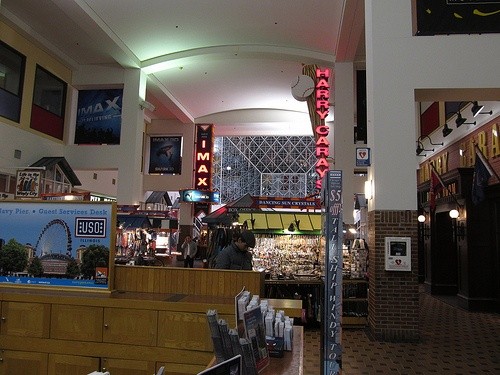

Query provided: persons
[181,235,197,268]
[214,230,255,270]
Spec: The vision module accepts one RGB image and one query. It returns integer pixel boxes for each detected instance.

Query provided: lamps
[455,102,492,128]
[288,223,296,232]
[242,219,254,227]
[417,215,425,223]
[442,112,476,138]
[118,221,130,231]
[471,101,484,117]
[232,214,239,225]
[416,135,443,155]
[449,210,459,219]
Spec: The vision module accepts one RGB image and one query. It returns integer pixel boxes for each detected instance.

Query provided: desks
[263,275,324,325]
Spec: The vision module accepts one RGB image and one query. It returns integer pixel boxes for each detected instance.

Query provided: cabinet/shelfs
[341,279,368,328]
